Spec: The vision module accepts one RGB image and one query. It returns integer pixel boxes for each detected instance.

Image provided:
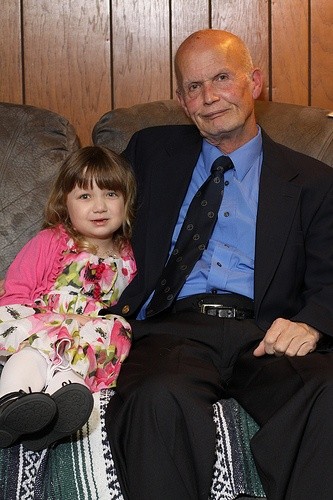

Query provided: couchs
[0,99,333,500]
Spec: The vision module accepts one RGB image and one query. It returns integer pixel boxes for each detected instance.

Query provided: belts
[136,290,254,319]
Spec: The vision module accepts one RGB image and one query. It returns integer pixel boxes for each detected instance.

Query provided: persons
[0,146,137,451]
[0,29,333,500]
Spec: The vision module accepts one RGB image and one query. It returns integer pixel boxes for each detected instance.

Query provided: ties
[145,155,234,319]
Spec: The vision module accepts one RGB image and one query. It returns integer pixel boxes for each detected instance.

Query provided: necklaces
[96,247,114,256]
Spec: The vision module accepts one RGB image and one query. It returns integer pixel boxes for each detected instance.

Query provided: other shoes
[0,385,57,448]
[20,380,94,451]
